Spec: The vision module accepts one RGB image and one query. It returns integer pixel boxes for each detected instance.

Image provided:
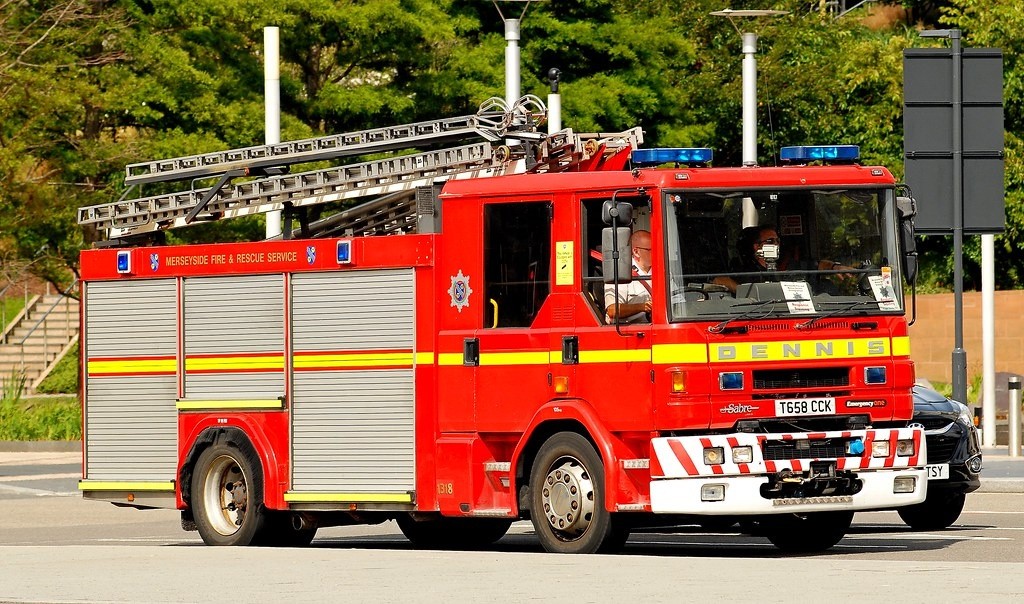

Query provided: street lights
[919,29,966,406]
[711,7,793,229]
[493,0,529,145]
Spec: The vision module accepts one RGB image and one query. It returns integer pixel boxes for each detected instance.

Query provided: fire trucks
[77,67,928,553]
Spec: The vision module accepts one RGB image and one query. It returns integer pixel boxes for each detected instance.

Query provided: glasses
[761,238,781,244]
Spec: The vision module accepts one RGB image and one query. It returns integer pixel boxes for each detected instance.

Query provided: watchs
[831,262,842,270]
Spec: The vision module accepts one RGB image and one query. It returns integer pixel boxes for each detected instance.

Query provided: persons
[602,230,652,326]
[711,227,857,300]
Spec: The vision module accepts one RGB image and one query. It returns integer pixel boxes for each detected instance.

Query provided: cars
[738,385,981,533]
